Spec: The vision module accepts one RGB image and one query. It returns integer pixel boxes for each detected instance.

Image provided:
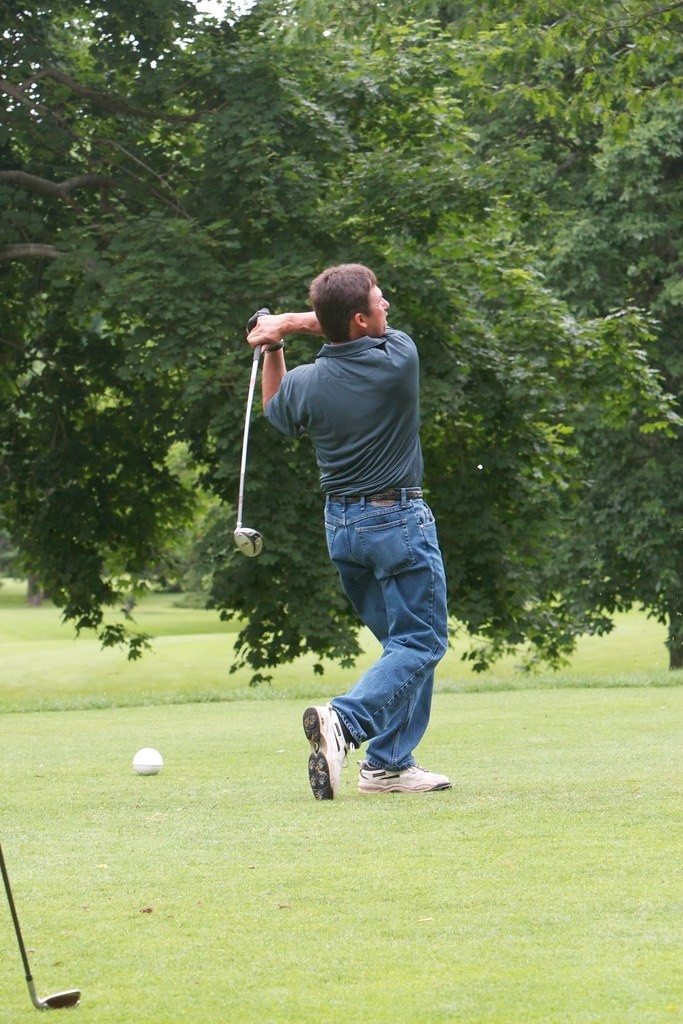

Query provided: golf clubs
[233,307,270,558]
[0,842,81,1011]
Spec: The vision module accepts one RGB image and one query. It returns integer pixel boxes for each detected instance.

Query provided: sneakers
[303,705,356,801]
[356,760,451,793]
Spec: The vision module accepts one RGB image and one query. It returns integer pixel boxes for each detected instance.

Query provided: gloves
[245,308,285,352]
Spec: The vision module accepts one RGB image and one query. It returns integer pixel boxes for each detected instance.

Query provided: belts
[329,490,423,503]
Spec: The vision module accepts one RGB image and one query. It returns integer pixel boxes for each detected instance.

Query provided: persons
[246,263,452,799]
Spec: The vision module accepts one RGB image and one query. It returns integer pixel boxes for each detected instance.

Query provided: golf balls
[132,747,164,776]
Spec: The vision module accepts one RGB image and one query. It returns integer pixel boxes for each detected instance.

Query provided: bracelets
[267,340,284,352]
